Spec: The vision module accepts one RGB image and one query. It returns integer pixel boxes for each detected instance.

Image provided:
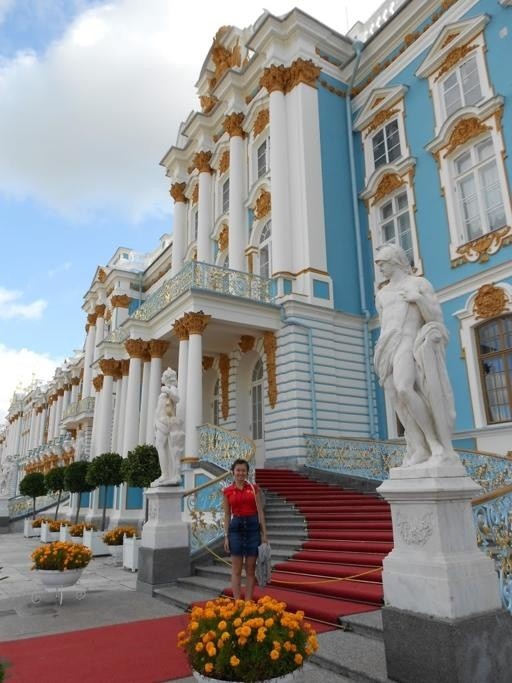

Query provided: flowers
[31,517,97,536]
[100,525,140,545]
[30,539,94,570]
[175,593,320,683]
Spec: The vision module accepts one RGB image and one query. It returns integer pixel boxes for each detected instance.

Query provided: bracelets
[224,533,229,536]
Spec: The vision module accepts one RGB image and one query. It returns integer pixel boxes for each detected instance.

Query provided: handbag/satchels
[257,543,271,586]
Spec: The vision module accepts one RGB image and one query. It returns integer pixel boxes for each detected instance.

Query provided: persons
[222,456,268,602]
[371,242,446,466]
[166,414,184,484]
[153,365,178,483]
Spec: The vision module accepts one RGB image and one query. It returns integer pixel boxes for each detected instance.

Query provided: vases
[30,571,89,607]
[109,545,125,565]
[189,661,305,683]
